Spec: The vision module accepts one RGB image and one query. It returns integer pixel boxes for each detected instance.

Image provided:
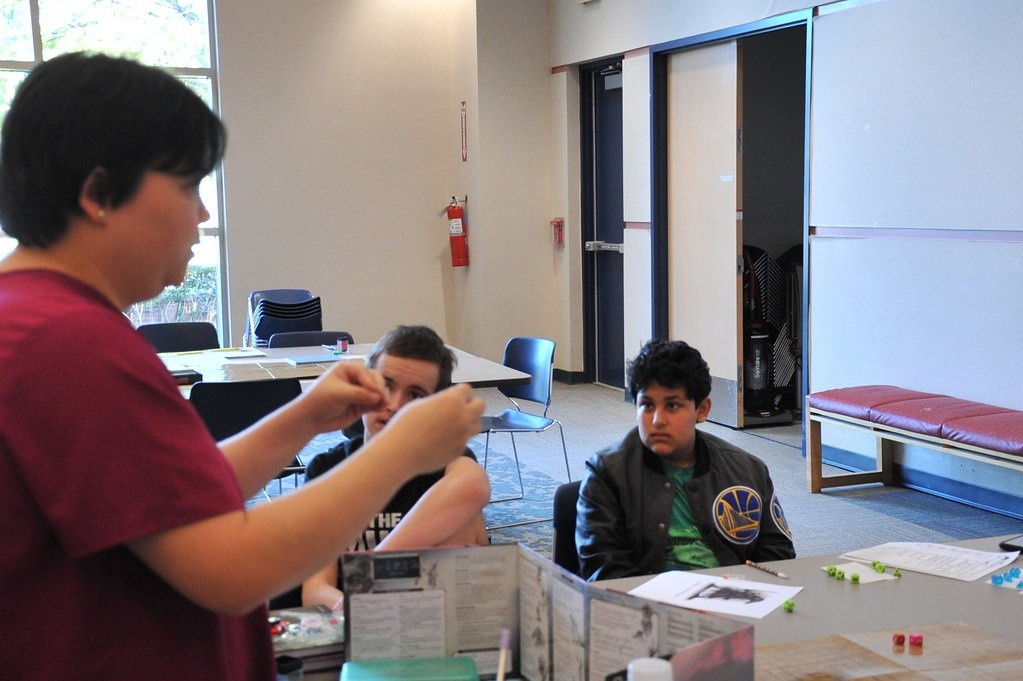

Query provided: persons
[0,50,484,681]
[575,340,796,581]
[301,325,491,611]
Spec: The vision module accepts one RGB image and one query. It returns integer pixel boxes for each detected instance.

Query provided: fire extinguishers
[438,196,469,267]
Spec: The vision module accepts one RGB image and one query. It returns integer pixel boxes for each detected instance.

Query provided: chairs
[478,336,572,531]
[135,321,220,354]
[243,288,354,348]
[189,379,308,496]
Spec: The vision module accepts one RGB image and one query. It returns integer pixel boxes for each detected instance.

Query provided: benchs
[804,385,1023,497]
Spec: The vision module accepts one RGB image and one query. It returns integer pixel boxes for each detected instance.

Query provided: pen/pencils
[321,344,335,350]
[746,560,789,579]
[496,629,511,681]
[333,351,348,355]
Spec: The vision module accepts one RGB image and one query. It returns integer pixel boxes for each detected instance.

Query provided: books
[284,354,343,364]
[224,349,268,358]
[166,360,202,384]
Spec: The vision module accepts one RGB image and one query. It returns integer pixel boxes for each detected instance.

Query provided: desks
[585,532,1023,644]
[154,342,531,402]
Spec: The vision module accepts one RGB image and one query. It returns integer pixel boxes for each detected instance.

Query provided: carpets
[242,427,569,561]
